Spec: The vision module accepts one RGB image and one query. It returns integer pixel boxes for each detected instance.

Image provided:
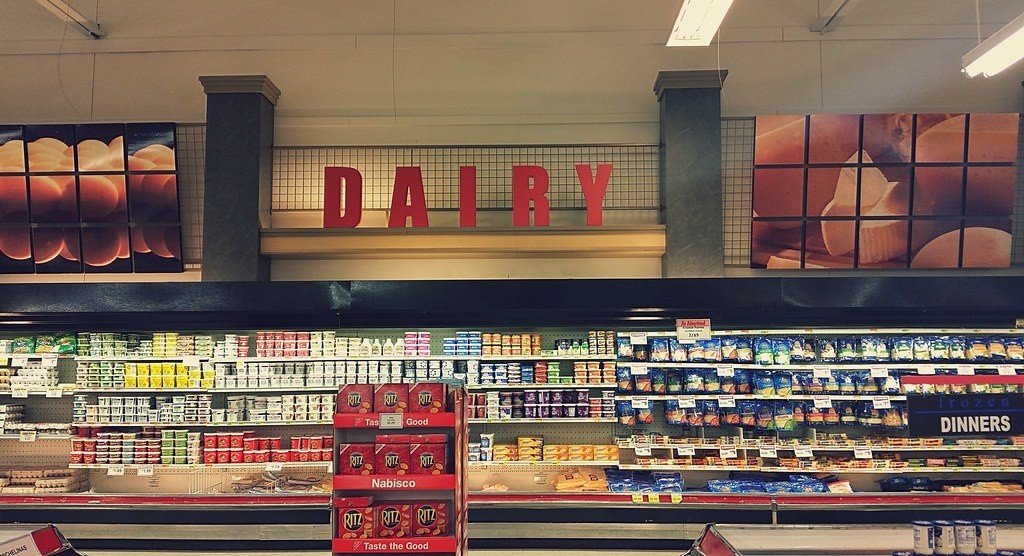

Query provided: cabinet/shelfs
[331,389,462,556]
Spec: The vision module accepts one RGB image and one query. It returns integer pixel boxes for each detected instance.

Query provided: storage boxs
[338,383,450,539]
[492,434,620,461]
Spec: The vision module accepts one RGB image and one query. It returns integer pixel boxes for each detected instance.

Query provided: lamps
[960,0,1024,78]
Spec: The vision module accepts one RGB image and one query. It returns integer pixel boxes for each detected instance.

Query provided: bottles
[360,338,405,355]
